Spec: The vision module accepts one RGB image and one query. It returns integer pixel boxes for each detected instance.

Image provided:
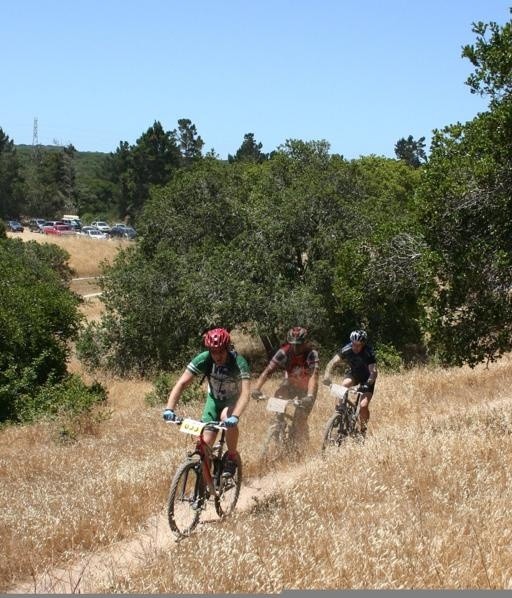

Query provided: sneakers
[222,460,236,478]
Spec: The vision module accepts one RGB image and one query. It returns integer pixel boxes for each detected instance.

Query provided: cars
[1,212,149,242]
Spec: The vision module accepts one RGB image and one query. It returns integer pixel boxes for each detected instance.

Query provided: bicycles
[248,390,307,483]
[320,376,366,460]
[167,411,245,539]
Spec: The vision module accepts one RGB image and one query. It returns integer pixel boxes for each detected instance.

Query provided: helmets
[350,330,367,343]
[205,329,229,350]
[287,327,307,345]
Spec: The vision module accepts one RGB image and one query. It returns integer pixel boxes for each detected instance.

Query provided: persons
[251,325,321,438]
[322,327,379,435]
[163,325,251,511]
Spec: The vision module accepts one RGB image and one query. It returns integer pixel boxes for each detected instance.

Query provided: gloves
[301,396,314,407]
[164,408,175,420]
[321,376,332,385]
[225,415,239,427]
[362,383,371,392]
[252,389,262,400]
